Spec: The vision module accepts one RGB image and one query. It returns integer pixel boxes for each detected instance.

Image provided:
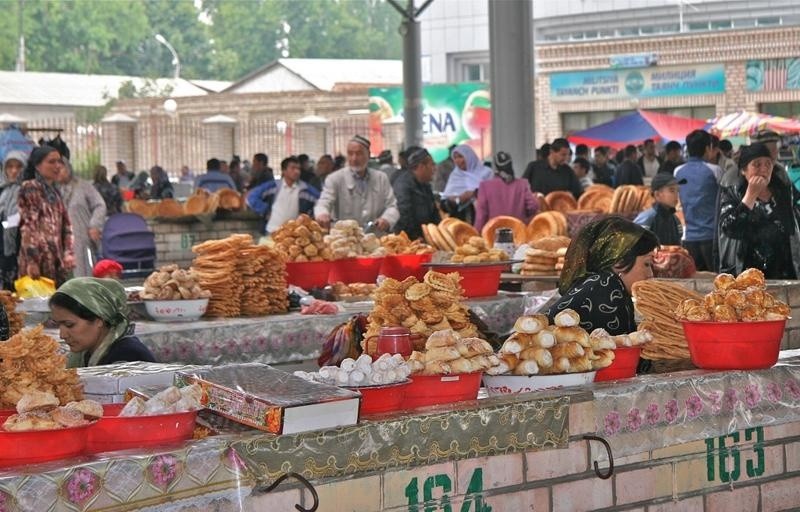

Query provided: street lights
[155,31,182,96]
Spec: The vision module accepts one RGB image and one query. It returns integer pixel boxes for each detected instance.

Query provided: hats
[651,172,687,191]
[750,129,780,145]
[407,149,428,166]
[348,134,370,149]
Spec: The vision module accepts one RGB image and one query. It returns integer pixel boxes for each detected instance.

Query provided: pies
[123,186,245,215]
[421,212,566,246]
[523,180,688,274]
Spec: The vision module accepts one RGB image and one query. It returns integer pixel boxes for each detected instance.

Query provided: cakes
[294,353,409,384]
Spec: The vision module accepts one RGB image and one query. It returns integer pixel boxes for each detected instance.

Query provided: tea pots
[365,326,426,363]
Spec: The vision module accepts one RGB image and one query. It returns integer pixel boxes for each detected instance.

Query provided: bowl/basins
[595,346,645,385]
[682,320,788,370]
[382,255,428,281]
[348,380,415,419]
[410,373,482,406]
[1,415,97,471]
[286,262,328,289]
[330,257,379,283]
[483,374,598,400]
[434,264,503,296]
[141,297,208,323]
[95,401,198,452]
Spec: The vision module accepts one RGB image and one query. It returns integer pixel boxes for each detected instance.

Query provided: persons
[49,277,155,366]
[1,126,797,291]
[540,216,660,376]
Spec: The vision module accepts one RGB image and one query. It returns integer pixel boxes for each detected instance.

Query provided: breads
[0,325,82,405]
[141,262,211,299]
[449,234,508,264]
[677,267,793,322]
[380,230,430,257]
[116,380,203,415]
[2,389,101,429]
[362,268,480,355]
[479,309,615,374]
[271,212,336,262]
[411,329,498,370]
[321,220,378,258]
[610,329,656,348]
